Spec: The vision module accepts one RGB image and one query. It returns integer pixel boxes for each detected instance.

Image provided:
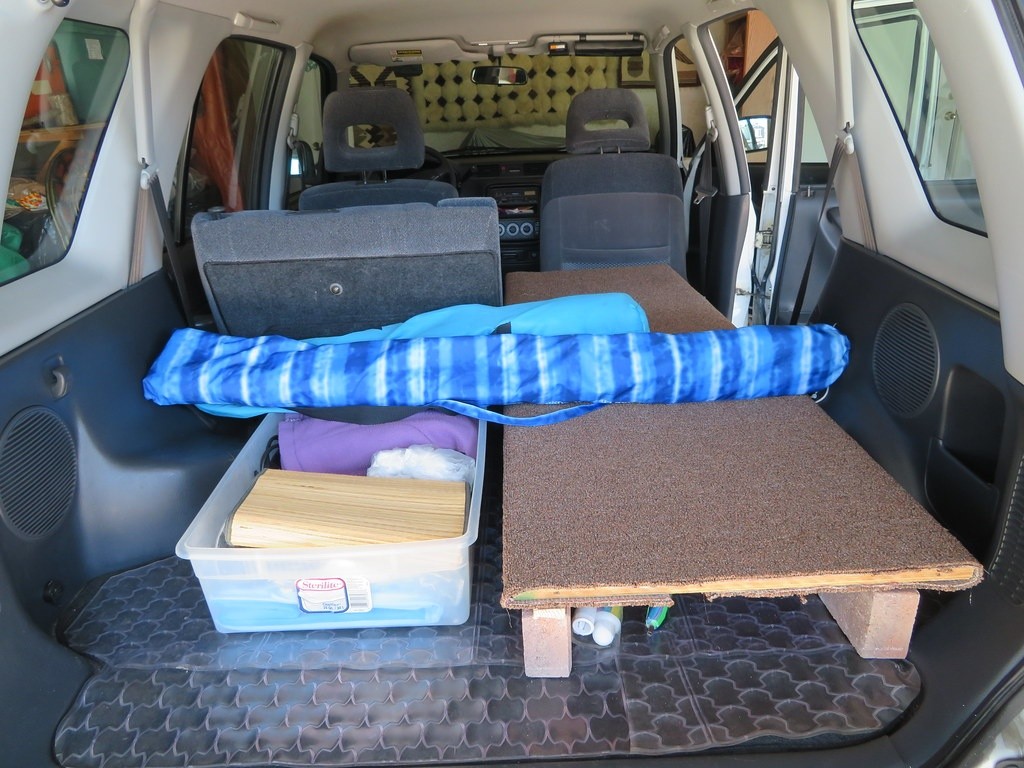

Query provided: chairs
[191,196,504,339]
[538,88,690,279]
[296,86,457,209]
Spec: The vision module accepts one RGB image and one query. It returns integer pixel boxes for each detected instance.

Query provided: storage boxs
[175,405,486,633]
